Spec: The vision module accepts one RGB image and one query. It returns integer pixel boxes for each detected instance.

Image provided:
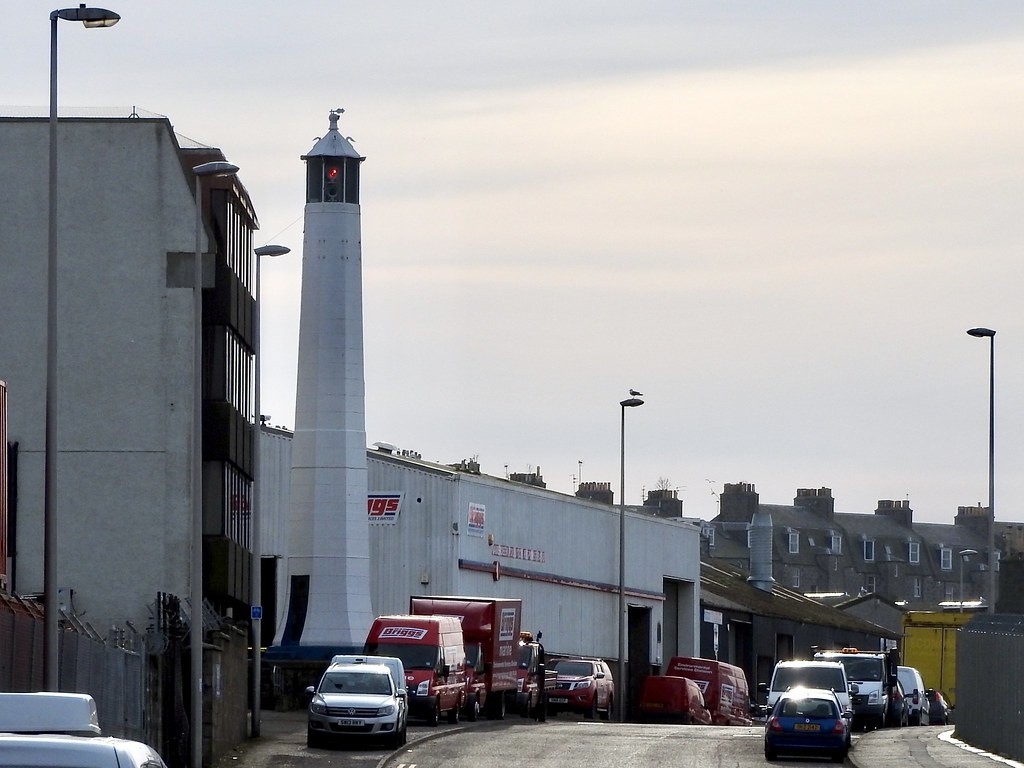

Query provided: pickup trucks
[307,656,409,751]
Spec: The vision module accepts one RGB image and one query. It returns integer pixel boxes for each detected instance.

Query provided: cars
[891,677,909,728]
[0,691,171,768]
[924,688,954,725]
[763,687,850,760]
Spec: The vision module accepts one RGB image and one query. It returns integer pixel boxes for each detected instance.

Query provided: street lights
[966,327,997,619]
[41,1,123,692]
[616,399,645,721]
[189,161,235,767]
[247,245,291,743]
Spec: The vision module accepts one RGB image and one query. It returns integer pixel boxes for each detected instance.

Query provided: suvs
[545,657,615,722]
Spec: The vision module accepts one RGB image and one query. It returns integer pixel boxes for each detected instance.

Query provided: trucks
[811,645,891,731]
[408,594,522,724]
[519,632,559,723]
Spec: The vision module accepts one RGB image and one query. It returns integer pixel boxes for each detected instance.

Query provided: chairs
[783,702,798,716]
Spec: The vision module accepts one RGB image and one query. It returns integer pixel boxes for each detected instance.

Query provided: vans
[641,677,712,725]
[665,656,754,727]
[362,615,468,726]
[767,661,852,731]
[896,665,931,727]
